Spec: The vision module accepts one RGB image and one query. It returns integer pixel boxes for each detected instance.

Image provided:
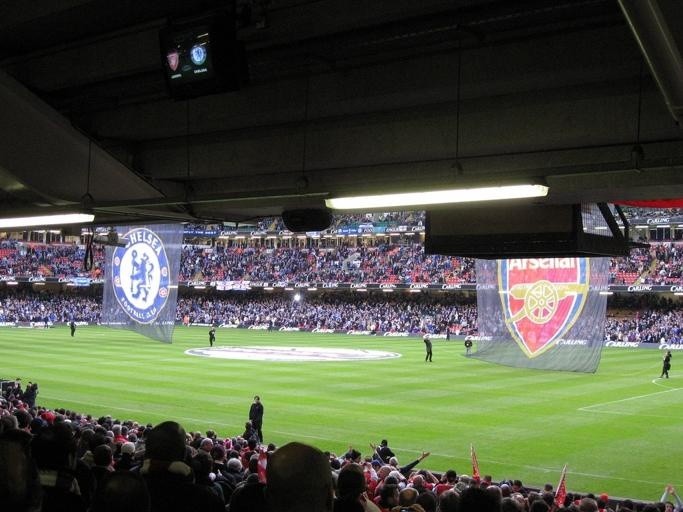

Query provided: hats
[93,444,113,466]
[350,449,361,461]
[120,442,136,456]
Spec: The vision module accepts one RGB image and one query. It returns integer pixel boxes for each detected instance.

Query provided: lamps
[0,207,94,232]
[322,174,552,211]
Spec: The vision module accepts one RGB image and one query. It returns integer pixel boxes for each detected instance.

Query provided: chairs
[0,420,507,511]
